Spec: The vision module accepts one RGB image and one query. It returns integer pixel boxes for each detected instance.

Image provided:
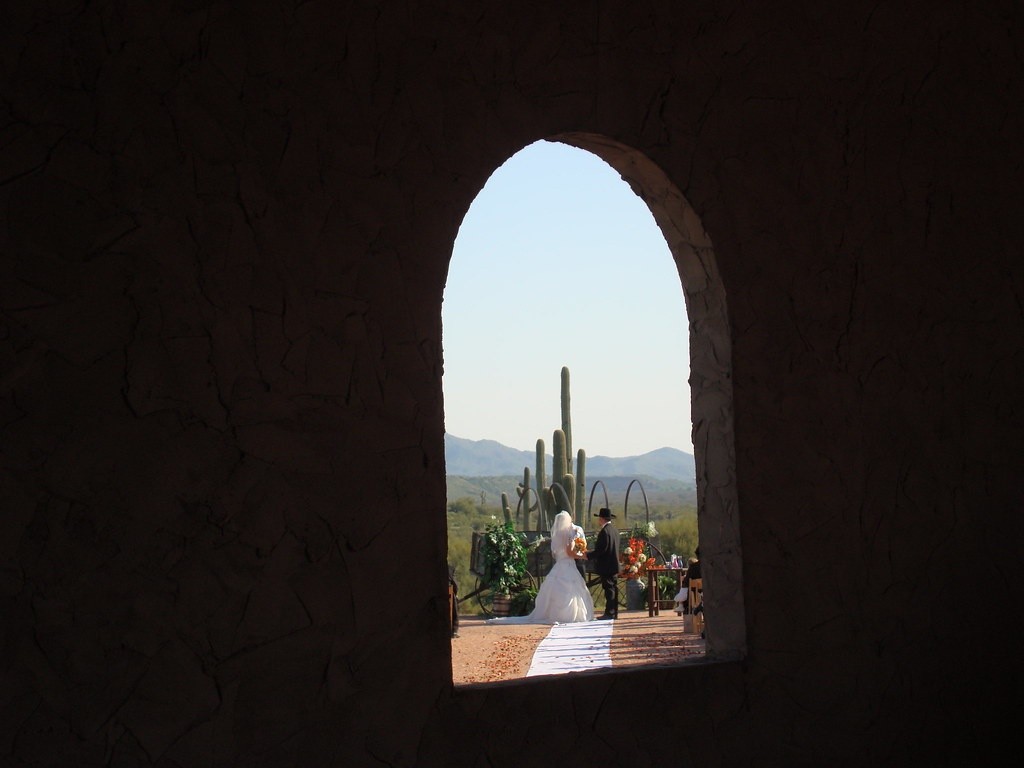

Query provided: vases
[625,573,646,612]
[492,588,515,616]
[665,554,684,570]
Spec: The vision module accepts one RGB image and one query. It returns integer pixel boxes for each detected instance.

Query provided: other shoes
[452,633,460,638]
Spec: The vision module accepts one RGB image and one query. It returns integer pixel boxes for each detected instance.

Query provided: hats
[593,508,617,519]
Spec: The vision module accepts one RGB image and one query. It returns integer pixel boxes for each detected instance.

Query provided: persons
[449,565,460,638]
[582,508,620,621]
[674,587,689,611]
[534,511,595,621]
[572,525,587,579]
[684,546,702,613]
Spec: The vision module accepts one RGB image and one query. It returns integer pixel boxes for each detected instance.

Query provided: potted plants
[666,577,678,610]
[659,575,669,609]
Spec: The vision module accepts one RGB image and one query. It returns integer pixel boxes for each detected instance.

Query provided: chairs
[688,578,705,636]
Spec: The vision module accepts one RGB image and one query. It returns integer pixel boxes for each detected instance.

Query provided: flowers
[574,537,588,556]
[616,521,659,581]
[483,514,549,600]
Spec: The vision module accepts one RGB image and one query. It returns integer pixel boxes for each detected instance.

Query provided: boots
[693,603,703,615]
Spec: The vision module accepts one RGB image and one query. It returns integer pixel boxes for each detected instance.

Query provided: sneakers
[673,607,685,612]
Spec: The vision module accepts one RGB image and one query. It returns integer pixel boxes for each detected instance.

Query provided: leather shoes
[596,613,618,620]
